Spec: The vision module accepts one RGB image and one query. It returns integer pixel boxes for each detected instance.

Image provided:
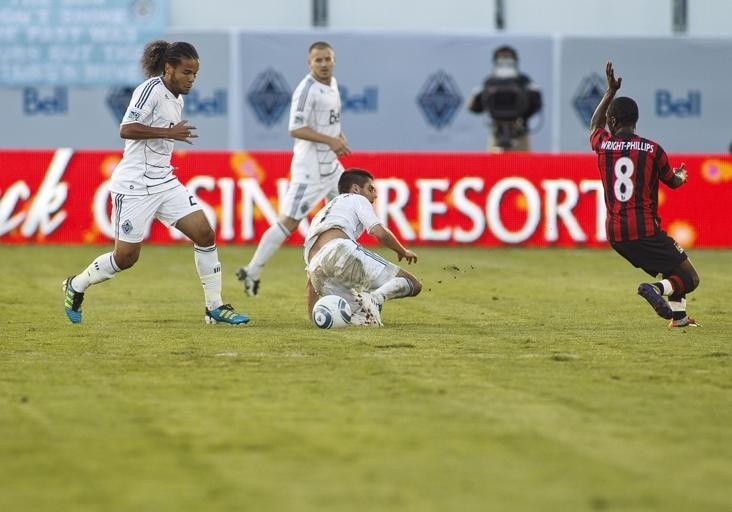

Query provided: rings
[189,133,191,138]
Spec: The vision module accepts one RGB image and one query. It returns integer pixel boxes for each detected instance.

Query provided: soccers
[312,296,352,330]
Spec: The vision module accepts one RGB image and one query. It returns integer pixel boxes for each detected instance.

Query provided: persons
[589,59,703,330]
[302,167,423,329]
[470,45,542,153]
[62,41,251,327]
[234,41,352,298]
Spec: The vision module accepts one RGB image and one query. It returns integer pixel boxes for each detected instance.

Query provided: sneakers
[638,283,703,327]
[205,304,249,326]
[235,267,259,296]
[62,275,84,323]
[356,292,384,327]
